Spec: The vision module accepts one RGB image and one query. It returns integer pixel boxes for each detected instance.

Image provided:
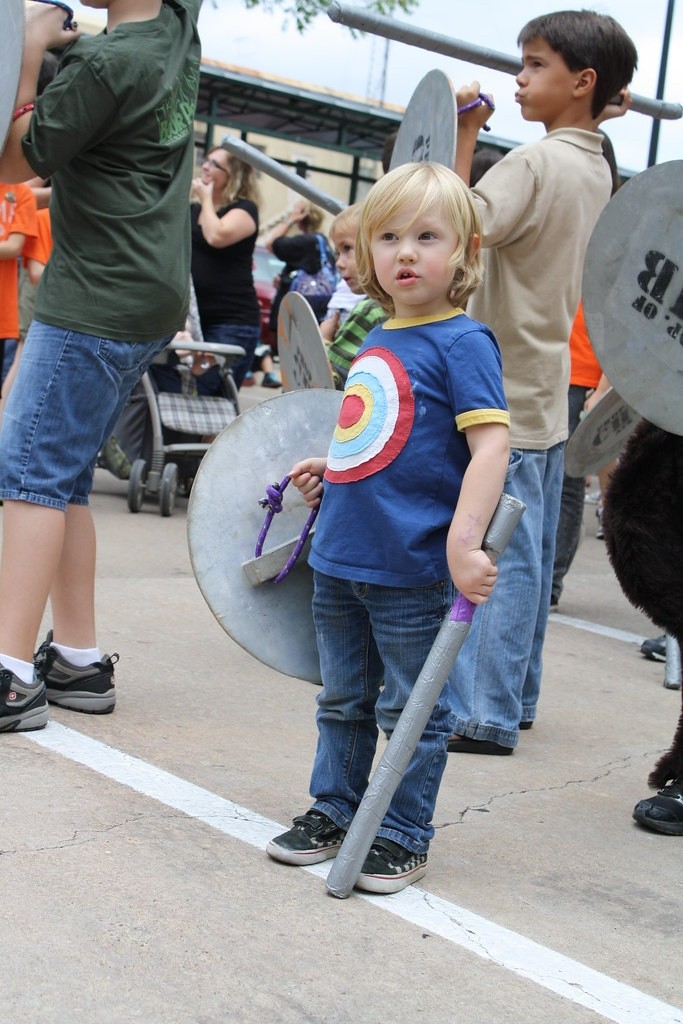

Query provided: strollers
[129,273,244,519]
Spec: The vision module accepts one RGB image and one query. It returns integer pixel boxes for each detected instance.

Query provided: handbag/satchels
[289,235,337,311]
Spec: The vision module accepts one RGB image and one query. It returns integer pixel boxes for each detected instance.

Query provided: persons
[328,201,391,389]
[243,344,284,389]
[266,160,510,894]
[0,176,52,413]
[448,11,636,755]
[267,203,339,358]
[0,0,261,733]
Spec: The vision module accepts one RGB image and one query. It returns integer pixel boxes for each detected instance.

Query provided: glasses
[204,158,230,177]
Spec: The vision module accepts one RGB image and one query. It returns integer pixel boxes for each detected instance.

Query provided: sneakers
[266,808,347,865]
[632,778,683,835]
[33,629,120,714]
[0,662,49,731]
[354,837,428,894]
[640,634,666,662]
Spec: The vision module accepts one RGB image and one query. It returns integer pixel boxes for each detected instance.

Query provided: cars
[251,244,290,342]
